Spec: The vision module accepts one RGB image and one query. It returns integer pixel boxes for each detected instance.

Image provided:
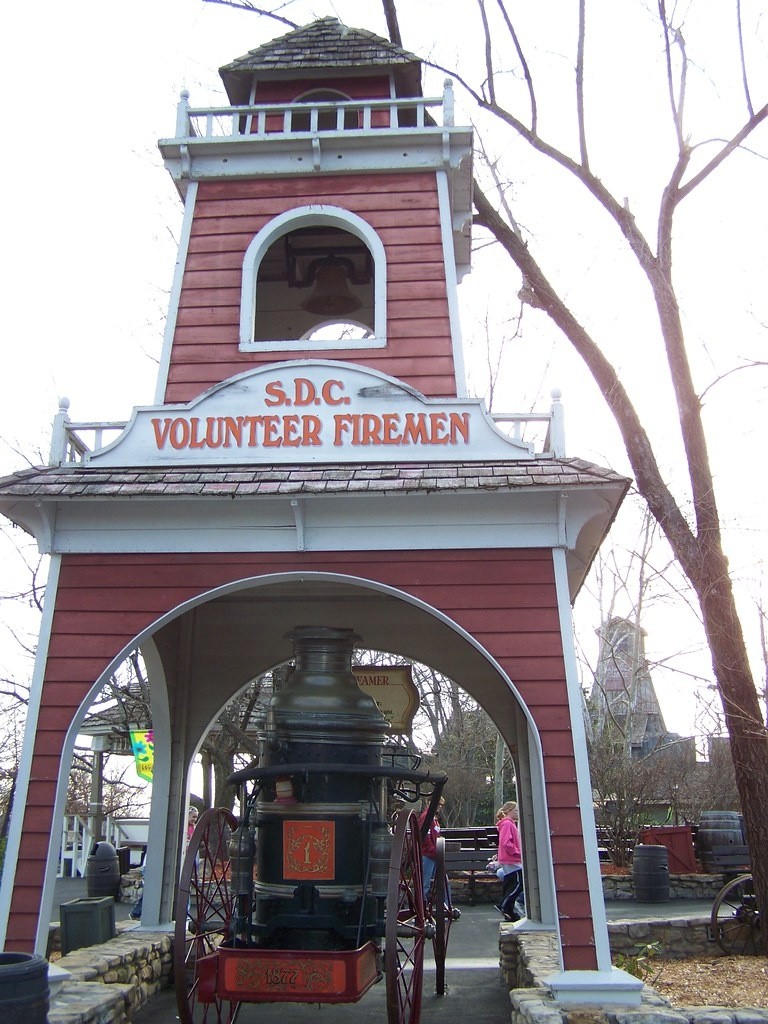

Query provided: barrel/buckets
[633,845,670,904]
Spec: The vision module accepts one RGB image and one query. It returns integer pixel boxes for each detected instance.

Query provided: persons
[493,801,524,921]
[419,795,453,909]
[128,805,198,920]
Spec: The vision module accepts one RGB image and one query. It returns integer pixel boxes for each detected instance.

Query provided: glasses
[189,808,196,815]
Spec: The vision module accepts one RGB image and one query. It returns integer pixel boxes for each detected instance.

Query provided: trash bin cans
[632,843,672,903]
[84,840,121,902]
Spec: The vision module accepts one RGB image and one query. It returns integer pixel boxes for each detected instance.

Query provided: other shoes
[494,904,518,921]
